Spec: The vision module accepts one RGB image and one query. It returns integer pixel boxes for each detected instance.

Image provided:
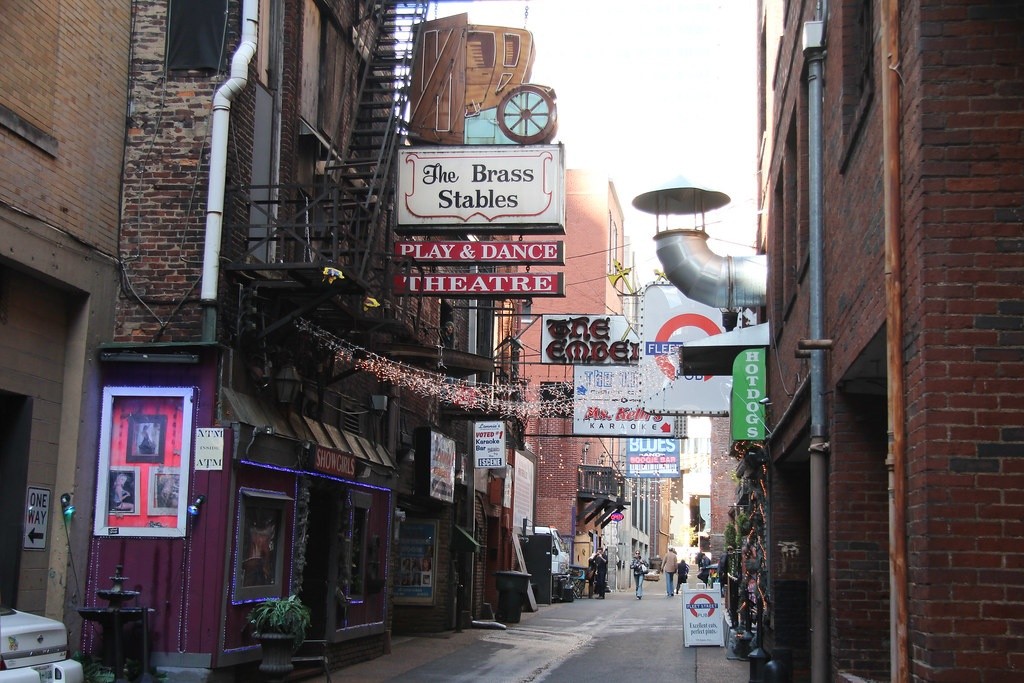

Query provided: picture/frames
[146,465,183,517]
[107,467,141,515]
[125,414,167,465]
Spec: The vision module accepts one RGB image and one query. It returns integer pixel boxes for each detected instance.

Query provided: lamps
[188,494,206,515]
[263,424,277,434]
[61,493,77,518]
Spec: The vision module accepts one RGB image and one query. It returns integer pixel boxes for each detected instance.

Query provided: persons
[661,545,677,596]
[138,425,156,454]
[394,558,430,587]
[593,548,609,599]
[441,322,454,348]
[160,478,178,507]
[717,546,734,598]
[675,559,690,594]
[112,473,134,512]
[697,553,711,589]
[630,550,649,599]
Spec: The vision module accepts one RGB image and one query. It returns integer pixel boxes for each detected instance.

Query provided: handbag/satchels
[642,564,648,574]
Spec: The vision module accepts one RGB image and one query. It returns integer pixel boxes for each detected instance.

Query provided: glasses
[635,553,640,554]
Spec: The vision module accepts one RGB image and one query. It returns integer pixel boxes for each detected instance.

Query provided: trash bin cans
[493,571,532,622]
[706,564,718,587]
[650,558,662,572]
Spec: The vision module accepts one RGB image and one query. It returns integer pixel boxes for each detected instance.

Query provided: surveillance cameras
[759,397,769,404]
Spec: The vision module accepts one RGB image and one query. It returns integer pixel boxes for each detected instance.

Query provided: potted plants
[245,595,312,672]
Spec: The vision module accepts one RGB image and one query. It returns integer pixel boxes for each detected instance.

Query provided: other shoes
[595,596,604,599]
[671,593,674,596]
[637,596,642,599]
